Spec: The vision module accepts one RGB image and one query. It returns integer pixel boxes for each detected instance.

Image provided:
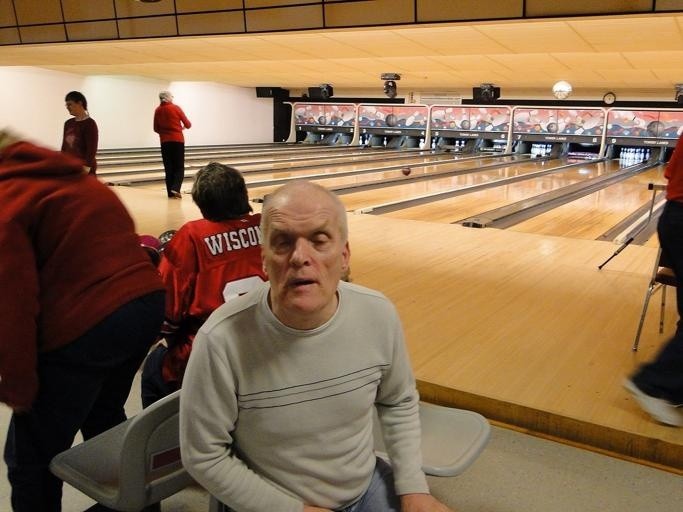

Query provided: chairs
[633,247,677,351]
[48,388,200,511]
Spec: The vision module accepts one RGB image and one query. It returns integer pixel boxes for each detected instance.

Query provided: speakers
[308,86,333,98]
[256,87,280,97]
[473,87,500,100]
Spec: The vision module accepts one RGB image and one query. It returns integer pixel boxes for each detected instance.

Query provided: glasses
[64,102,77,106]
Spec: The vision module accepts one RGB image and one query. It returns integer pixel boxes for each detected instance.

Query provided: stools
[370,397,490,477]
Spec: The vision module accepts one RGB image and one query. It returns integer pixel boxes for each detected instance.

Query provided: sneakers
[168,187,182,199]
[622,366,683,431]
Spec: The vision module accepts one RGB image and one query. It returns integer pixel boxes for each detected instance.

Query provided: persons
[621,127,682,428]
[139,161,269,412]
[56,91,99,179]
[0,124,164,512]
[150,87,191,203]
[177,179,455,511]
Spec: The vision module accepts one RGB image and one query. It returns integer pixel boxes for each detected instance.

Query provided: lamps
[552,81,572,99]
